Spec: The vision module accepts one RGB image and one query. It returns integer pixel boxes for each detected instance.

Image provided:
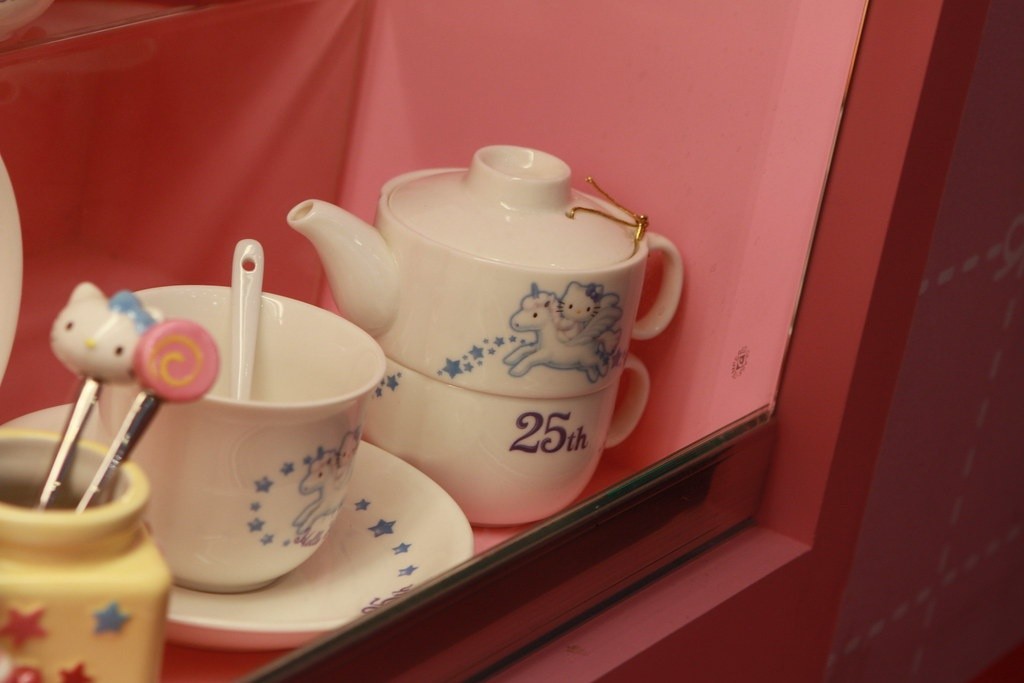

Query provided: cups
[97,279,385,594]
[359,349,651,528]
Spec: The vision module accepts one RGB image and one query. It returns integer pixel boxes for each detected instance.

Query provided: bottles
[0,427,172,683]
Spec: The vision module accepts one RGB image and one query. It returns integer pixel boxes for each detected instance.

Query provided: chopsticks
[31,281,221,513]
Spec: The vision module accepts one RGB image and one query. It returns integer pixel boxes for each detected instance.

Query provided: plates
[0,402,475,651]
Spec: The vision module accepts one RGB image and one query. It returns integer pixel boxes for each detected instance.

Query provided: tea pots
[284,144,684,400]
